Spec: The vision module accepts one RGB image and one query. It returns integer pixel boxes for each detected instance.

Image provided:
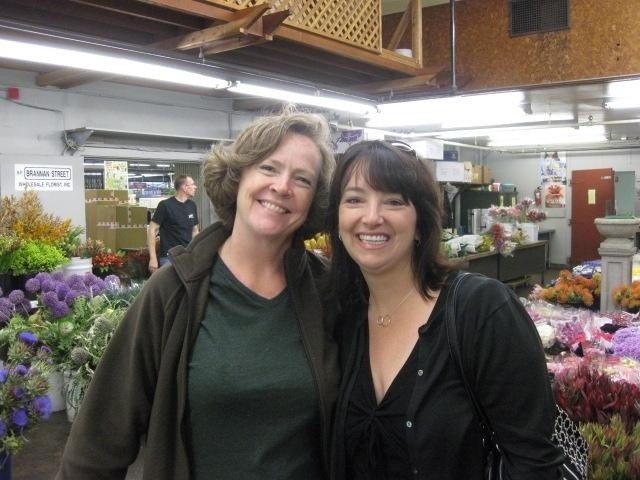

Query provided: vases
[60,256,94,280]
[518,223,539,242]
[497,222,514,239]
[62,370,95,423]
[30,366,66,413]
[0,271,41,299]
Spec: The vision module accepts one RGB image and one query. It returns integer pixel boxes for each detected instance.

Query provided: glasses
[184,183,195,187]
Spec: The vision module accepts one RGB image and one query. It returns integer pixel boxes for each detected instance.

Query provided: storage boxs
[86,189,129,203]
[117,224,148,250]
[85,197,118,224]
[86,222,119,253]
[116,200,148,227]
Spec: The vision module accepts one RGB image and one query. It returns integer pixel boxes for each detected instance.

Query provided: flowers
[550,364,639,435]
[0,183,73,244]
[0,332,62,479]
[487,202,519,224]
[545,355,639,387]
[518,259,640,361]
[575,413,640,480]
[439,229,456,258]
[73,235,130,282]
[304,231,332,260]
[0,234,72,300]
[489,220,504,243]
[0,270,122,365]
[513,199,547,225]
[473,237,492,252]
[49,284,145,420]
[495,238,521,258]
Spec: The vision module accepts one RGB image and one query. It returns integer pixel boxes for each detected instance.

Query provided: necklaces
[367,285,417,328]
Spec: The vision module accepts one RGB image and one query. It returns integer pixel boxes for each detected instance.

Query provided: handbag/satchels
[446,271,591,480]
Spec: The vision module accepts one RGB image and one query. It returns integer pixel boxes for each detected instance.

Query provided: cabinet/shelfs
[450,241,548,287]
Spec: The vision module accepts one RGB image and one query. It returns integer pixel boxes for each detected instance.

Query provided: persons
[148,173,199,273]
[326,140,565,480]
[56,106,338,479]
